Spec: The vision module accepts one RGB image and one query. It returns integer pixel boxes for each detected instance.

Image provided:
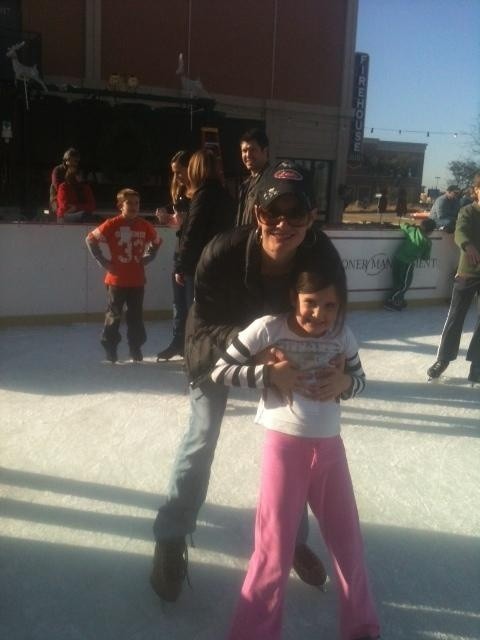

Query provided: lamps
[338,184,345,195]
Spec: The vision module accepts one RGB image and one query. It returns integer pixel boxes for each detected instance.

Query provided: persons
[381,219,438,313]
[86,190,161,364]
[426,172,480,383]
[209,258,380,639]
[460,187,474,207]
[49,148,104,224]
[148,159,346,603]
[429,186,457,233]
[155,129,273,362]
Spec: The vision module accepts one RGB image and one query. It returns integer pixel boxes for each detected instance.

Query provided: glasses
[255,207,310,227]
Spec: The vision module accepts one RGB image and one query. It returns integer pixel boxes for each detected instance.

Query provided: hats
[256,158,315,212]
[447,185,460,193]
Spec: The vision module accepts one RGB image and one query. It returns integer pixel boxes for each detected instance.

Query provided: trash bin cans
[378,198,388,213]
[396,199,407,217]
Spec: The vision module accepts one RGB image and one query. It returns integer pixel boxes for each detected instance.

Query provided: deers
[5,40,49,93]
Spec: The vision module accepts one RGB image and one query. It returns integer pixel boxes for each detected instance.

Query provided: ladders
[202,128,224,191]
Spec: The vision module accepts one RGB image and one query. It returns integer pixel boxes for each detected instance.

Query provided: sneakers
[151,537,187,601]
[384,300,407,311]
[428,360,449,377]
[129,347,142,361]
[468,364,480,383]
[105,347,118,361]
[292,544,326,585]
[157,340,184,359]
